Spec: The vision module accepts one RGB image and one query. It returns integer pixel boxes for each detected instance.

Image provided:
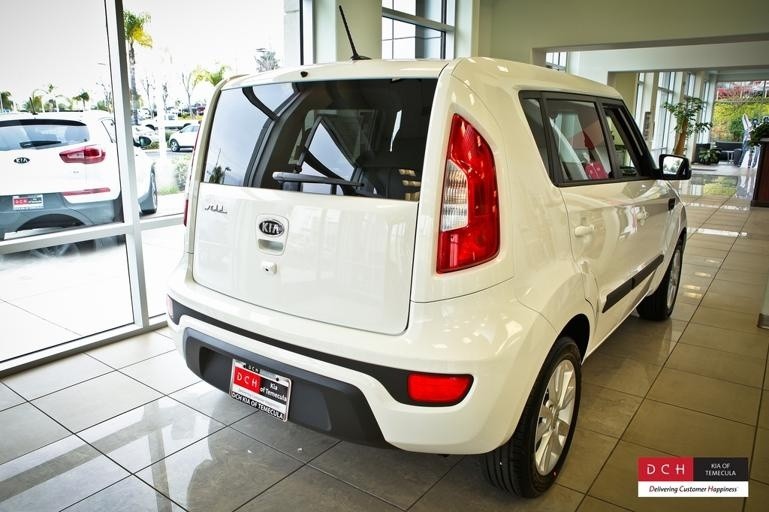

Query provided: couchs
[716,141,743,162]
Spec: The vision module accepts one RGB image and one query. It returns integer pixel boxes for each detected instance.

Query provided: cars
[141,115,199,130]
[169,125,200,151]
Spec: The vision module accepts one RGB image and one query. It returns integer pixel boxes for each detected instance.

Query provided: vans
[0,110,157,259]
[165,55,690,498]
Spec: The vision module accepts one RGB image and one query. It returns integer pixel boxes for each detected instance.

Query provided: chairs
[692,144,722,165]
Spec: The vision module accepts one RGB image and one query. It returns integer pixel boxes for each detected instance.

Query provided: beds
[722,151,734,164]
